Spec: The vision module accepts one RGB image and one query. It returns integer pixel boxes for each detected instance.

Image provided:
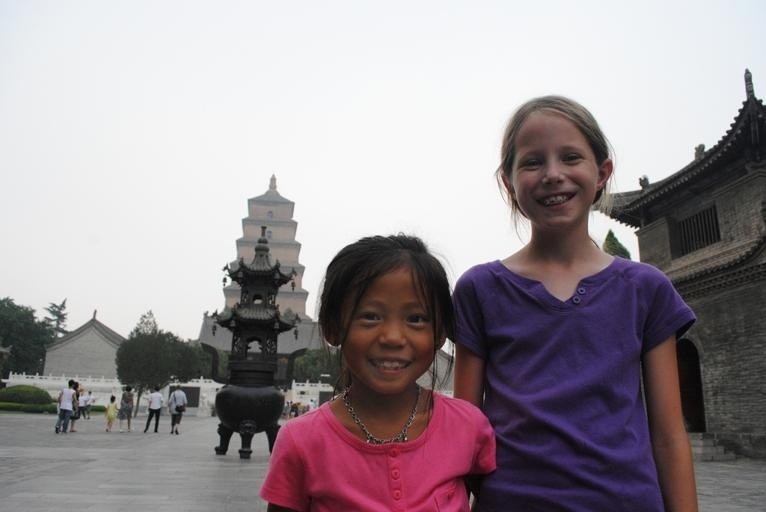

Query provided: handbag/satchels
[176,405,185,412]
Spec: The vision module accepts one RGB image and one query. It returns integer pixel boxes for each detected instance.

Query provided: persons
[143,385,164,433]
[258,234,498,512]
[54,379,96,434]
[281,398,318,420]
[450,94,699,512]
[167,384,188,436]
[104,395,120,433]
[116,385,134,433]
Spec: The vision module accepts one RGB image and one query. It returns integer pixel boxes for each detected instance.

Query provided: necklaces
[343,383,422,445]
